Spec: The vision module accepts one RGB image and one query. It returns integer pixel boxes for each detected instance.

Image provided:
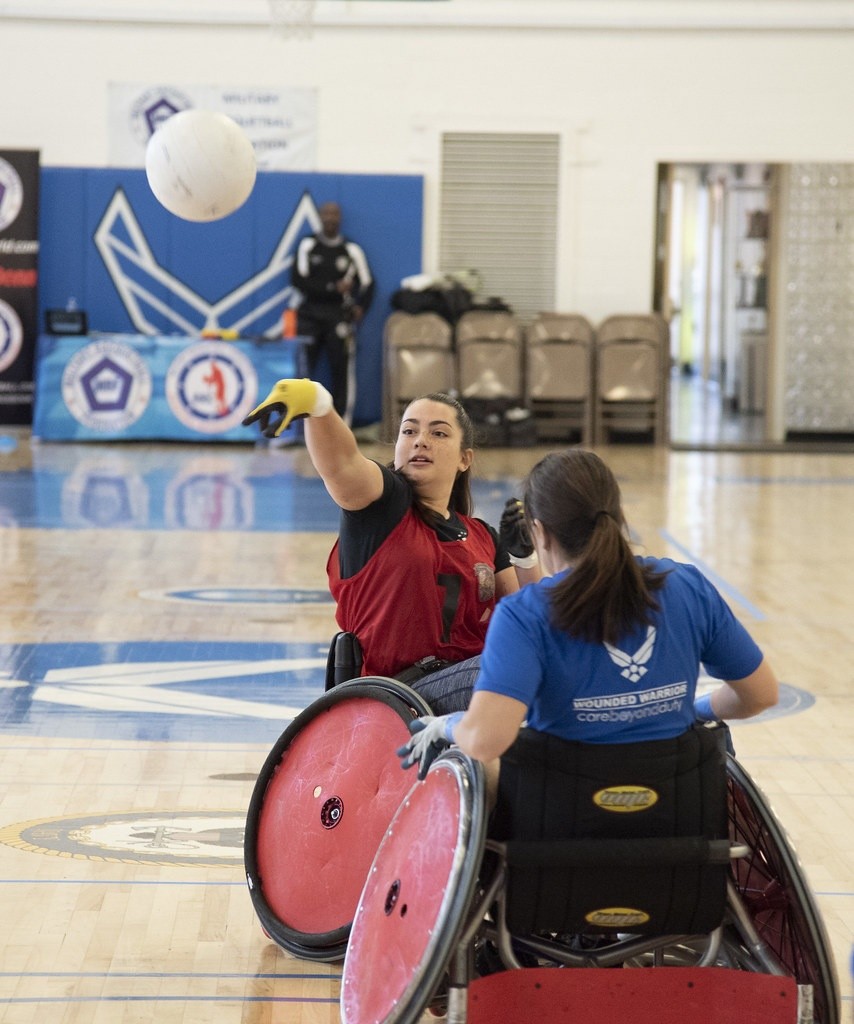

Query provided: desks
[35,334,315,447]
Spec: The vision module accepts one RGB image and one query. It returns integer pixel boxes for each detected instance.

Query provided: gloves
[499,497,538,569]
[240,378,333,438]
[397,710,462,780]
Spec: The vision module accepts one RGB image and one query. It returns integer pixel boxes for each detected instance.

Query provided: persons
[277,201,375,448]
[241,379,542,715]
[396,447,778,973]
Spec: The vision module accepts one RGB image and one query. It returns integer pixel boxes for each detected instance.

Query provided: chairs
[387,307,670,447]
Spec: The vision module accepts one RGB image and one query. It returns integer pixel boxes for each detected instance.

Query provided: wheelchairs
[339,724,844,1024]
[243,631,441,961]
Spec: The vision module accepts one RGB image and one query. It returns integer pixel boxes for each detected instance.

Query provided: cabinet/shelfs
[727,181,765,408]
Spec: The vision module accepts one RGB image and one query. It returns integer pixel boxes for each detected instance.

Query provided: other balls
[151,107,256,221]
